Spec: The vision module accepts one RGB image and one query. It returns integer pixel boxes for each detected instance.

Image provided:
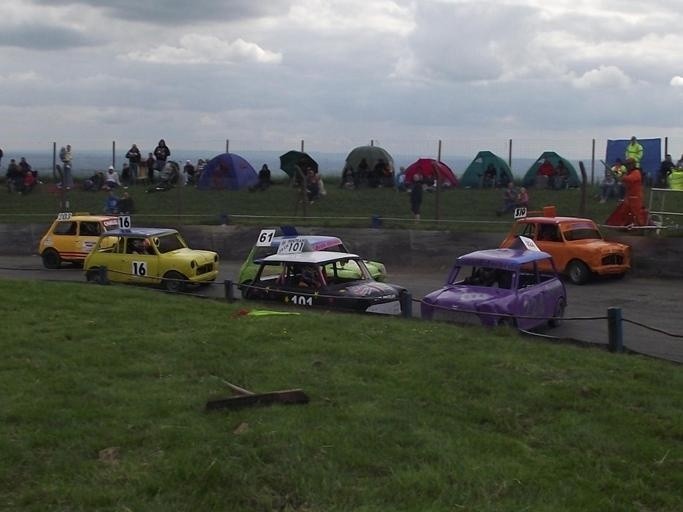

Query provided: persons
[477,267,499,288]
[131,240,150,255]
[341,158,440,222]
[299,265,321,289]
[101,139,174,215]
[483,158,568,215]
[598,137,683,227]
[60,145,72,190]
[183,158,207,186]
[5,156,38,194]
[258,164,271,191]
[297,156,321,203]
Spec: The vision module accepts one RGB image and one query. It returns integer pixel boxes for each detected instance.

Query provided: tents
[198,153,258,189]
[346,146,580,186]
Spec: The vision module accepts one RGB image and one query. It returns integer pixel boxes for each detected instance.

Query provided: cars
[418,245,567,335]
[38,212,126,267]
[501,206,629,283]
[236,233,387,292]
[84,227,219,293]
[244,251,406,317]
